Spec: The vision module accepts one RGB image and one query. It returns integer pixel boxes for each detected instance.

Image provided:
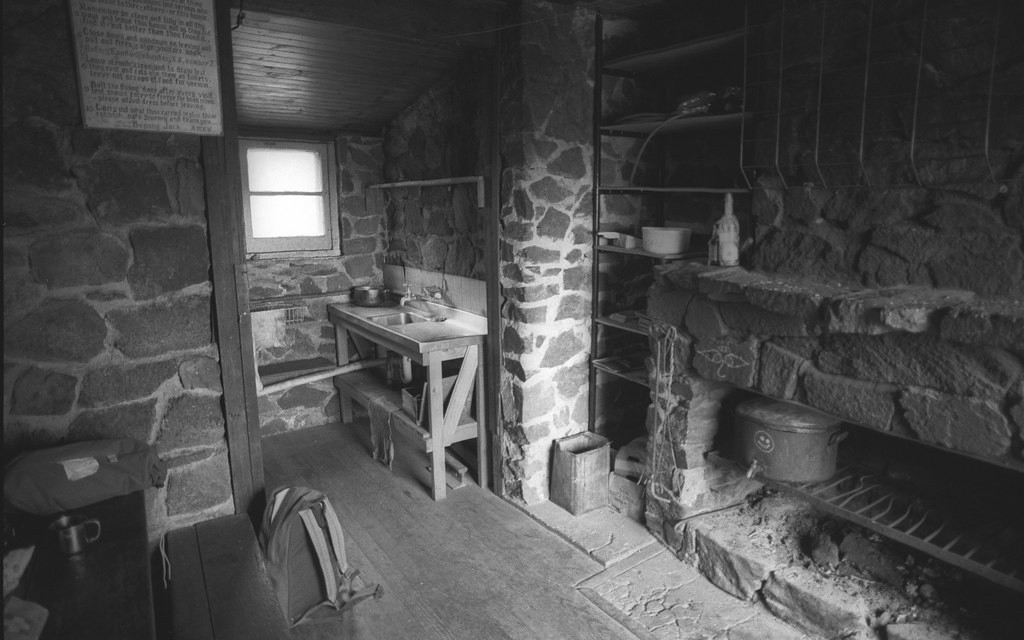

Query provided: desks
[0,488,159,640]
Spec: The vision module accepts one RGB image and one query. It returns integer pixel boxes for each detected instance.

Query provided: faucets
[397,282,423,307]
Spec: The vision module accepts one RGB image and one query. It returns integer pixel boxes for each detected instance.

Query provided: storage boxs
[401,384,426,426]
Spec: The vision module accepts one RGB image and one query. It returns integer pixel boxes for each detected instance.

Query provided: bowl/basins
[641,226,691,255]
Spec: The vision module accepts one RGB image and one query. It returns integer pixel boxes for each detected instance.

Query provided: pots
[719,396,850,482]
[352,285,393,307]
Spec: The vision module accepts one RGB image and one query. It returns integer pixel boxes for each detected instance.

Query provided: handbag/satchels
[0,437,166,516]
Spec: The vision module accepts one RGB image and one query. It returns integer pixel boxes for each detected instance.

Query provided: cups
[48,514,102,555]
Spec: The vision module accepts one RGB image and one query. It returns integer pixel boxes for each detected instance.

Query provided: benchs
[164,509,293,640]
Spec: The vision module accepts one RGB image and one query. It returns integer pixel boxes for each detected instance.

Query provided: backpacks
[258,484,384,629]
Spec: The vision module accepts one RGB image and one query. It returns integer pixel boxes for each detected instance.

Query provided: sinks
[365,310,430,327]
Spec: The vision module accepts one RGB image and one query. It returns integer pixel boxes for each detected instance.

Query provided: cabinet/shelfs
[587,0,758,473]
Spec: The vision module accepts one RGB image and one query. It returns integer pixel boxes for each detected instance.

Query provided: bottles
[707,192,740,267]
